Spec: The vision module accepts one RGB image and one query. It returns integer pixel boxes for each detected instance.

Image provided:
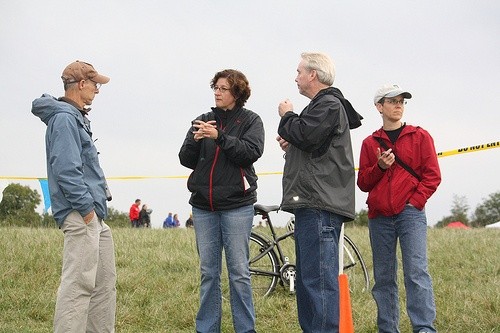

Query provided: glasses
[84,81,102,90]
[212,84,231,92]
[383,99,407,105]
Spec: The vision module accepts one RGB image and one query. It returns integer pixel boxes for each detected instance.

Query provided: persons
[356,84,442,333]
[31,60,117,333]
[178,69,265,333]
[174,214,180,228]
[130,199,141,227]
[139,204,150,227]
[185,213,193,227]
[275,52,364,333]
[163,213,176,228]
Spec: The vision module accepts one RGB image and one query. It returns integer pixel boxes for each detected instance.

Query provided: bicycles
[221,204,369,303]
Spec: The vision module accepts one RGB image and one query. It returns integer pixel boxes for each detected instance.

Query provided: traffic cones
[338,273,354,333]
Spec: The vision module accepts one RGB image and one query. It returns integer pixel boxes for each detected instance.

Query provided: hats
[61,60,111,84]
[373,83,412,104]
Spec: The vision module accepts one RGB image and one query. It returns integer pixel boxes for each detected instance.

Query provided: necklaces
[400,121,403,126]
